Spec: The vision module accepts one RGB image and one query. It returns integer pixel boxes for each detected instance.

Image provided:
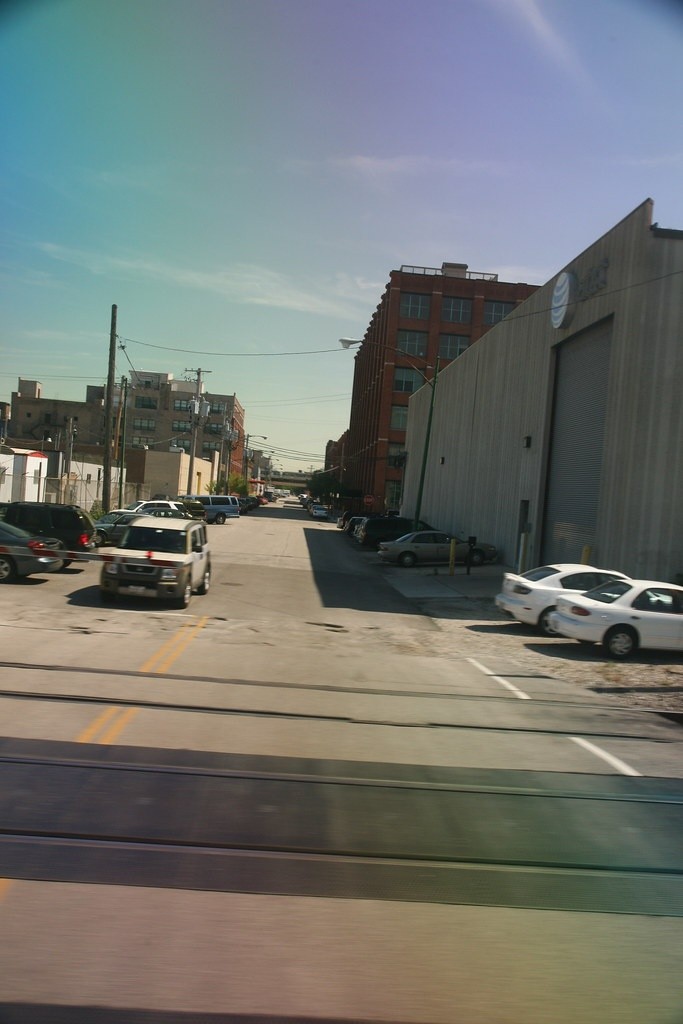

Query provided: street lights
[246,434,270,483]
[338,337,441,527]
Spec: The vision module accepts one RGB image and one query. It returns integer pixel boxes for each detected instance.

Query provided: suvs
[0,500,98,568]
[98,517,213,608]
[109,500,193,520]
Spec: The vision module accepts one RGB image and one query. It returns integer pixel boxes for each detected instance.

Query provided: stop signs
[364,495,372,506]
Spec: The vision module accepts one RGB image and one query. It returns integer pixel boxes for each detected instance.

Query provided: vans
[181,495,241,525]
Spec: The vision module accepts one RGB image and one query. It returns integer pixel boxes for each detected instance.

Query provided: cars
[138,507,184,521]
[0,522,66,584]
[494,563,675,637]
[92,512,157,547]
[352,515,441,547]
[547,579,683,659]
[298,493,328,517]
[376,529,498,568]
[336,508,386,537]
[237,494,289,516]
[180,500,207,523]
